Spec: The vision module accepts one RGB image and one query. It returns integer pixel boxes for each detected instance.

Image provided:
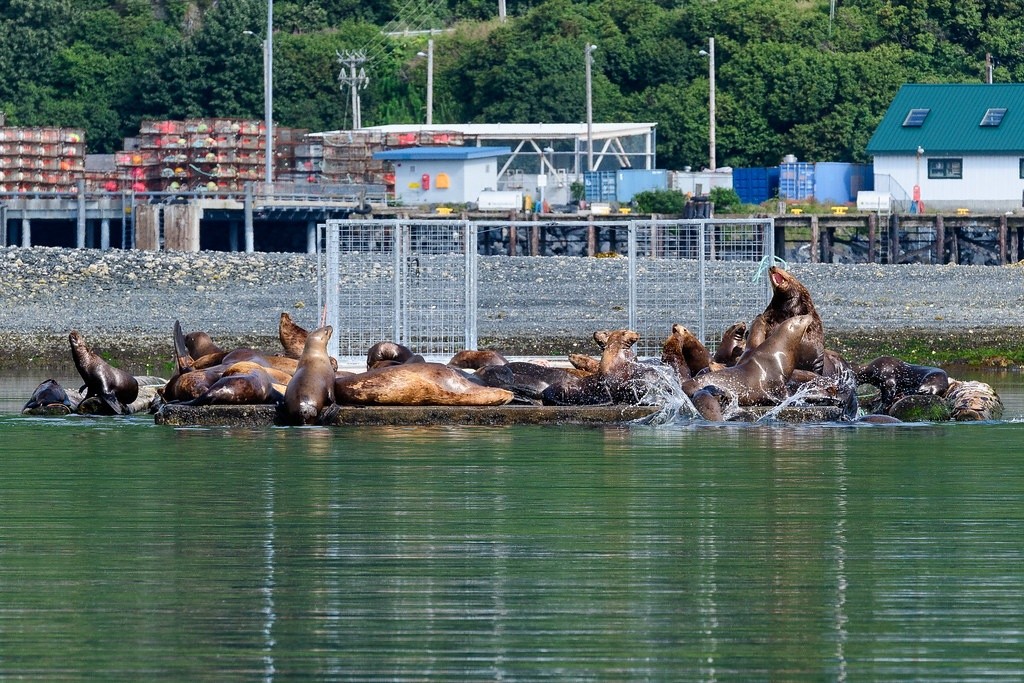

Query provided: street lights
[584,44,598,170]
[243,31,272,201]
[698,49,716,171]
[339,80,357,130]
[417,52,433,124]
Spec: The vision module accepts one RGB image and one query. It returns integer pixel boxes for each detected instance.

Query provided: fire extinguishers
[913,184,920,202]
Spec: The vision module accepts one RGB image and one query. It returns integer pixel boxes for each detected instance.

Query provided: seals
[19,264,1006,420]
